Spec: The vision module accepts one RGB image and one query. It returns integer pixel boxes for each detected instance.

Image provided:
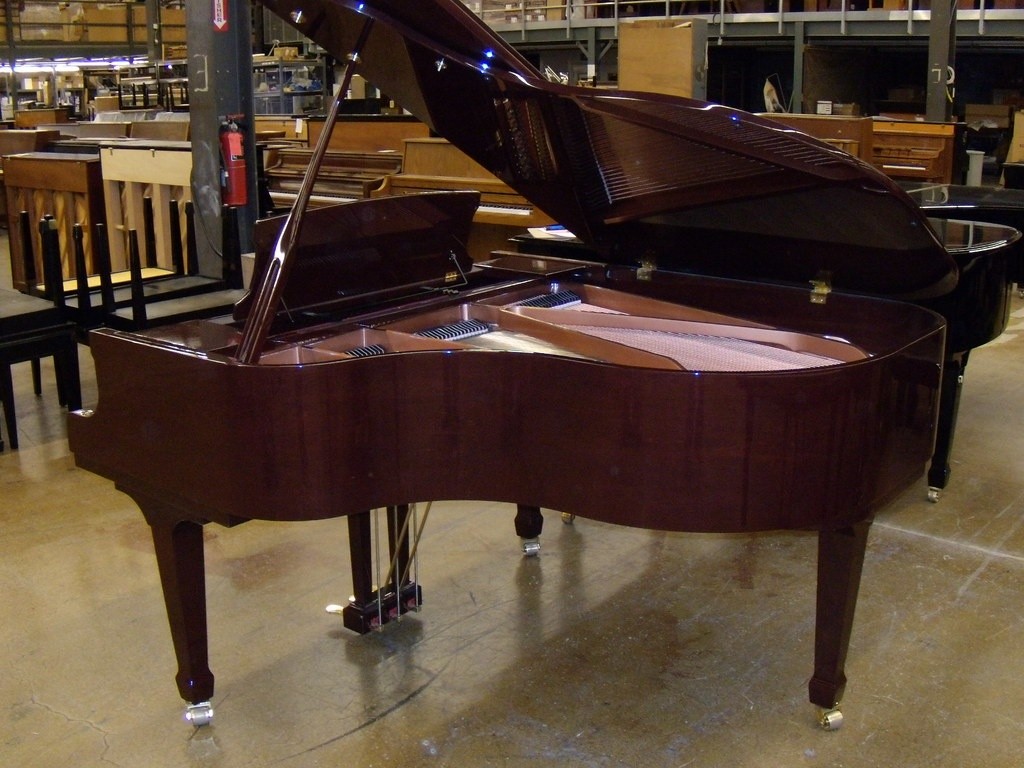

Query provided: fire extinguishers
[217,114,249,208]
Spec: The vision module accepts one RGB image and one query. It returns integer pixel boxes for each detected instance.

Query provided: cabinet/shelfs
[254,54,327,117]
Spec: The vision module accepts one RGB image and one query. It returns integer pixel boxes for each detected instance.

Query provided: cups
[965,150,985,186]
[274,47,298,60]
[251,53,265,62]
[919,182,948,205]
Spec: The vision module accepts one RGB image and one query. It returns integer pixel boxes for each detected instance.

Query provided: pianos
[65,1,947,731]
[370,2,1023,502]
[2,106,1024,316]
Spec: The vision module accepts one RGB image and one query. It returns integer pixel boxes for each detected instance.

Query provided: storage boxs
[460,0,566,23]
[5,101,34,119]
[274,48,298,61]
[888,88,914,100]
[833,103,860,114]
[351,75,376,99]
[994,87,1021,108]
[964,104,1011,129]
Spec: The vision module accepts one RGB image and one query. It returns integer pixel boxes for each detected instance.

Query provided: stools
[0,196,272,451]
[118,82,190,112]
